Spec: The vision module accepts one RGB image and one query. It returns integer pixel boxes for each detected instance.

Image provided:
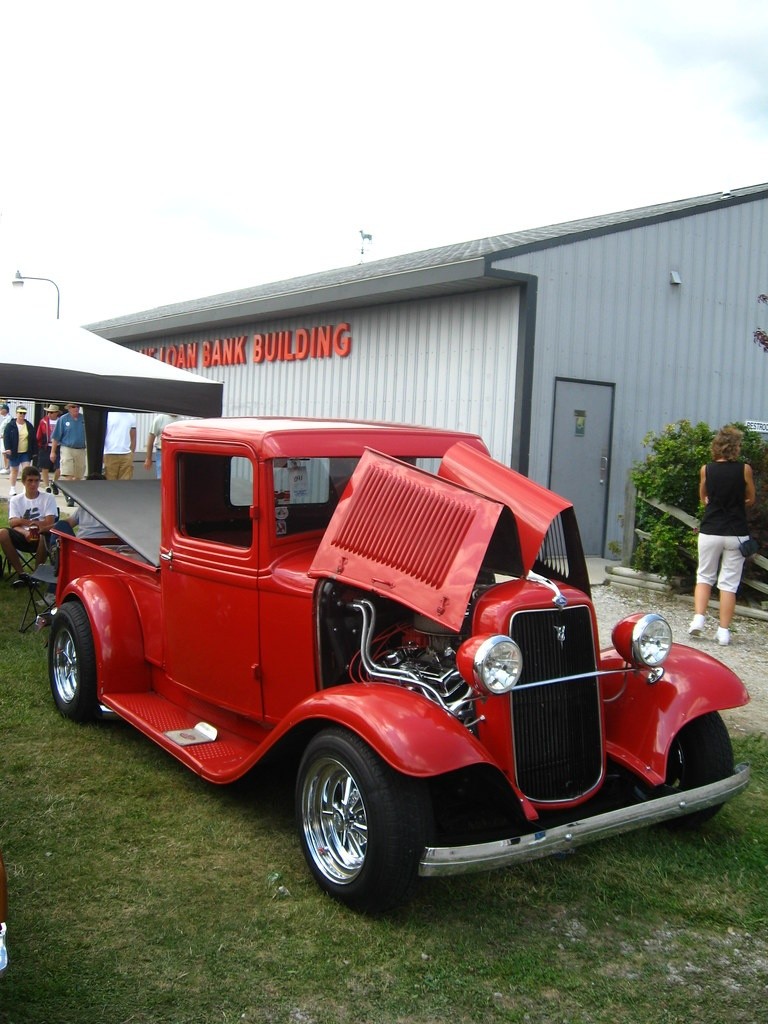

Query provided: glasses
[20,412,26,415]
[72,406,79,408]
[47,411,57,414]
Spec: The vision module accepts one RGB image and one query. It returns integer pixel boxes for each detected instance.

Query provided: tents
[0,308,224,418]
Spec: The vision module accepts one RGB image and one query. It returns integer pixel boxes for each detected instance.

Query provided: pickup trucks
[48,417,755,917]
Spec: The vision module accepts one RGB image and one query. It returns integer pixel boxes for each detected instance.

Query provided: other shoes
[11,574,30,588]
[67,495,74,507]
[50,481,59,495]
[46,487,51,493]
[0,468,10,474]
[31,578,44,587]
[9,488,17,496]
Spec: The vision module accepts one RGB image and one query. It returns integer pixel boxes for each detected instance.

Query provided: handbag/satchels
[739,537,760,557]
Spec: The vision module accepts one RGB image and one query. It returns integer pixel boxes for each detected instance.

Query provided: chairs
[1,507,60,582]
[18,564,58,633]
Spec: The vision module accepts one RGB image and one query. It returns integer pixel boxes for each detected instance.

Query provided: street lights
[10,269,61,321]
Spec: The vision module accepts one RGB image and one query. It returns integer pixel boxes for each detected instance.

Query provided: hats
[44,405,63,415]
[16,408,27,412]
[0,404,9,409]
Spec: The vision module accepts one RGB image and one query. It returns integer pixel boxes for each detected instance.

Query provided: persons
[688,425,755,645]
[144,415,182,479]
[103,412,137,480]
[0,403,87,507]
[35,472,115,607]
[0,466,58,588]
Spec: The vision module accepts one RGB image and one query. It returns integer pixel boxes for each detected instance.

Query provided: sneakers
[36,592,55,609]
[688,614,705,637]
[714,626,730,646]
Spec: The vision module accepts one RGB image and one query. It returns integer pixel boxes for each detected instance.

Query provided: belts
[74,447,84,450]
